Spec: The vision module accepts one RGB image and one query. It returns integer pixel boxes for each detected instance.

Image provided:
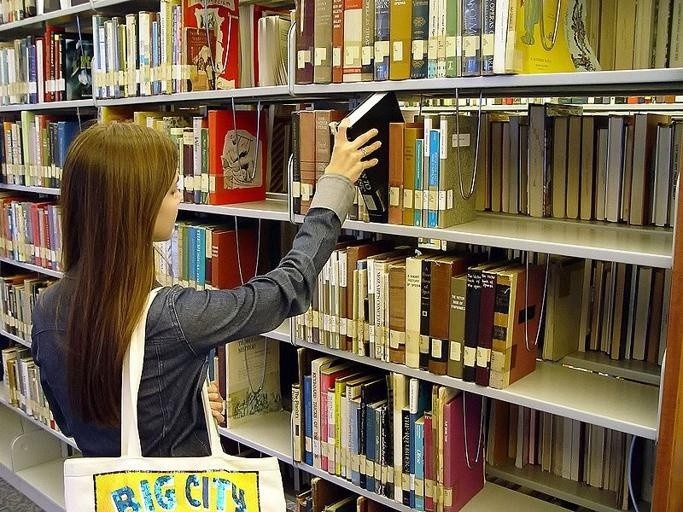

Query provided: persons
[30,120,381,458]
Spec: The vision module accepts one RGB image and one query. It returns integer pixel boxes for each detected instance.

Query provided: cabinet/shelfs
[4,3,683,512]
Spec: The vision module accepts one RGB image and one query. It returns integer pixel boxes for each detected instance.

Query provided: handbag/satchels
[62,285,287,512]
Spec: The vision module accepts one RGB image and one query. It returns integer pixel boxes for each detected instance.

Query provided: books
[284,1,682,512]
[1,1,289,424]
